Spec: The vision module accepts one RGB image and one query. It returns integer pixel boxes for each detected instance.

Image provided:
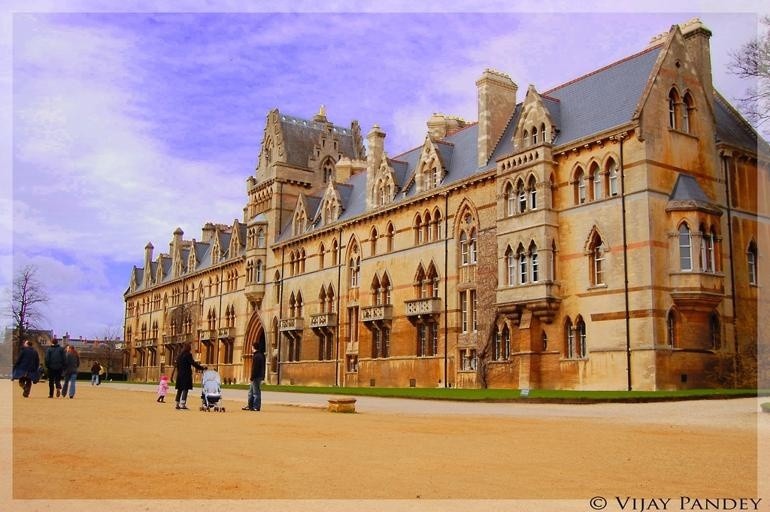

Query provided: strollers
[199,368,226,412]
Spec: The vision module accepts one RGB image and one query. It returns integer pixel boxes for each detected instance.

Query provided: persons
[175,342,207,408]
[61,344,79,399]
[10,340,41,397]
[156,375,169,402]
[44,338,64,398]
[37,362,44,374]
[241,342,266,410]
[90,361,101,385]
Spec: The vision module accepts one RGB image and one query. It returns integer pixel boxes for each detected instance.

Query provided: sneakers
[242,406,260,411]
[175,404,189,409]
[48,390,76,399]
[157,400,166,403]
